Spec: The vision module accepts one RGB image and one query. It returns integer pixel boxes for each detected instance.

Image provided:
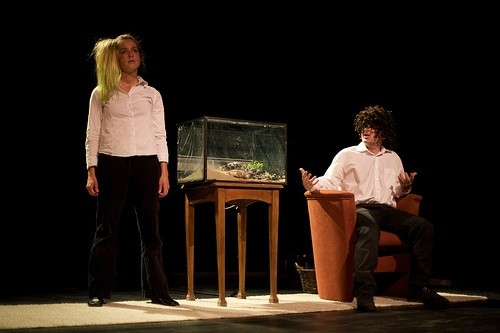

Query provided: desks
[181,182,284,307]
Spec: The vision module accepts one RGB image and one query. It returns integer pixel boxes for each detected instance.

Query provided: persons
[299,106,450,313]
[85,34,180,306]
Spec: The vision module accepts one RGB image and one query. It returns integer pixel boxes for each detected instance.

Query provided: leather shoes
[151,290,179,306]
[87,293,103,306]
[357,290,376,312]
[407,287,451,306]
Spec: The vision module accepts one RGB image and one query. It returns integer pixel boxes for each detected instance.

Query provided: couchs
[303,189,423,303]
